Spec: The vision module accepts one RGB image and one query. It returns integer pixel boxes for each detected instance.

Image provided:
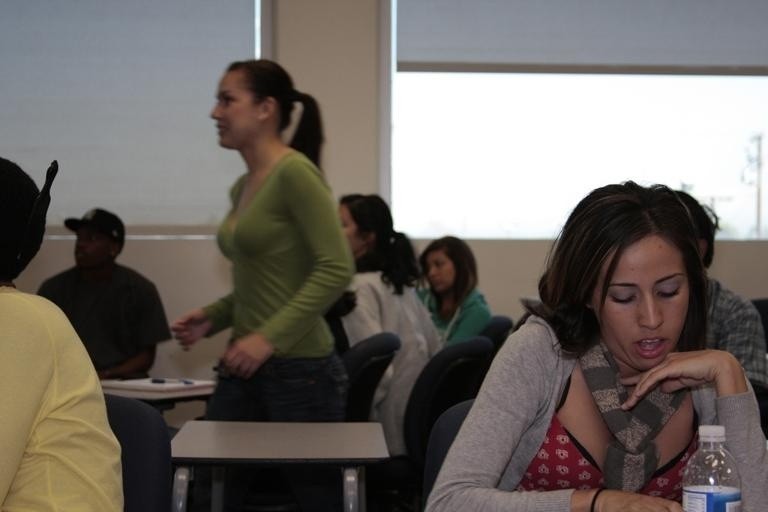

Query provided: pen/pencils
[151,379,193,384]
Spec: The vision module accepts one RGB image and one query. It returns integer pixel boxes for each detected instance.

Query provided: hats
[64,208,124,245]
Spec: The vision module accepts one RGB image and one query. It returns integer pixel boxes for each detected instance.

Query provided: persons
[169,58,358,510]
[1,155,126,512]
[339,194,443,462]
[674,190,768,441]
[425,181,768,512]
[416,237,490,346]
[37,209,171,380]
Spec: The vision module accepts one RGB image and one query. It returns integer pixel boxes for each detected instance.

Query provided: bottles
[680,423,743,512]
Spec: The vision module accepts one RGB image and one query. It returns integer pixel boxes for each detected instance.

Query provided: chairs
[97,295,767,512]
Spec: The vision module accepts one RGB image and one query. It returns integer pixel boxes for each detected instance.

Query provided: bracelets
[591,485,606,511]
